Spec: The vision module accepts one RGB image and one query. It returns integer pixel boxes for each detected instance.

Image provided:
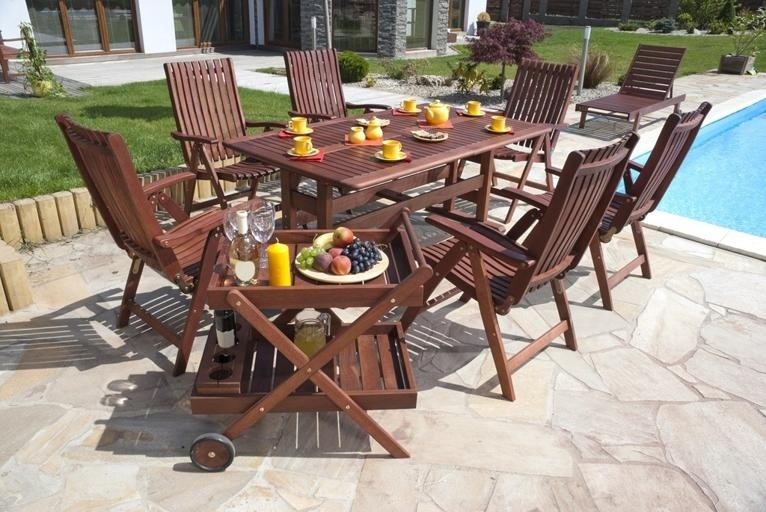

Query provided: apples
[312,248,351,276]
[333,227,356,248]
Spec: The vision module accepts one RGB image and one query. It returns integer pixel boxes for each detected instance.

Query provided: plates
[464,111,486,116]
[414,132,448,142]
[287,147,319,157]
[399,108,422,114]
[295,246,389,283]
[285,128,313,135]
[375,150,406,162]
[357,119,390,127]
[485,125,512,133]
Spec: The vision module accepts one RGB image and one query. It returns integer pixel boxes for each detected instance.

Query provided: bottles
[228,210,258,286]
[214,311,237,348]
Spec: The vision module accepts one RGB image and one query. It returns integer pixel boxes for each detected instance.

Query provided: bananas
[312,232,334,251]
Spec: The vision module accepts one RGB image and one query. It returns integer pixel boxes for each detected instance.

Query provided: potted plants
[719,14,765,75]
[18,26,62,98]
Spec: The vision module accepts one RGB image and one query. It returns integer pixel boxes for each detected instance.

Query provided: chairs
[161,57,280,213]
[402,133,640,400]
[284,49,390,215]
[53,115,268,377]
[530,101,711,311]
[453,60,576,222]
[573,43,685,131]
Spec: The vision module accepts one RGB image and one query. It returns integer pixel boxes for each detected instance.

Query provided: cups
[491,116,505,131]
[289,117,307,132]
[294,309,330,360]
[403,99,416,111]
[383,140,402,159]
[465,101,481,114]
[294,136,313,154]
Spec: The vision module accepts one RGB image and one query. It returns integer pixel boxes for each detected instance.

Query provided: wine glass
[223,202,250,242]
[251,201,274,267]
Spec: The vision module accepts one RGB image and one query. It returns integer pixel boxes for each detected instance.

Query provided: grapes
[297,247,324,269]
[342,241,383,274]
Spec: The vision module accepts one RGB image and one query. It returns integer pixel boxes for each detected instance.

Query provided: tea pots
[424,100,452,125]
